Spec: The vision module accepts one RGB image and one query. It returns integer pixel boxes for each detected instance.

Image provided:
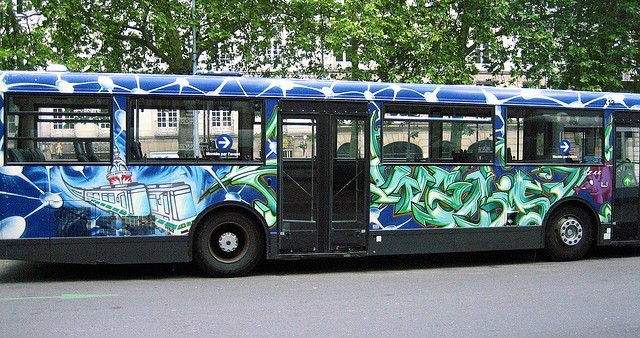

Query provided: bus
[1,69,639,276]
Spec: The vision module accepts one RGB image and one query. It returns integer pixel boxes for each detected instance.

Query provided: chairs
[9,147,46,162]
[73,139,94,162]
[464,149,477,162]
[134,141,143,159]
[452,149,463,162]
[565,151,578,163]
[85,139,101,162]
[212,141,223,160]
[414,157,420,162]
[208,140,211,151]
